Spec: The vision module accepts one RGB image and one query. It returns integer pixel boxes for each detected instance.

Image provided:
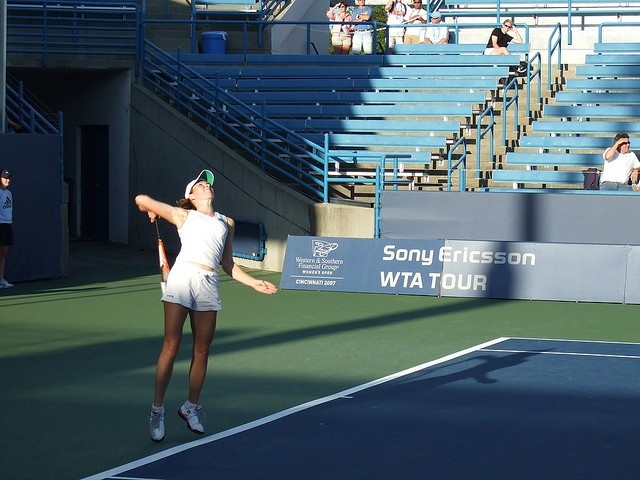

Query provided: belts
[356,28,370,31]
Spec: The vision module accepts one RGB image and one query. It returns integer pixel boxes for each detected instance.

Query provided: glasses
[505,24,513,28]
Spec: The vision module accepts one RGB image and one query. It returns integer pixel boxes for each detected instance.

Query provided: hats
[431,11,442,18]
[184,170,216,200]
[1,168,15,179]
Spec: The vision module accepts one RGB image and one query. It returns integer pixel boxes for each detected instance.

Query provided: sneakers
[176,400,205,435]
[149,403,166,443]
[0,279,14,289]
[517,66,526,73]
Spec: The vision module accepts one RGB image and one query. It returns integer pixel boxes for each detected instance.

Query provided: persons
[598,132,640,191]
[418,12,449,45]
[483,19,523,56]
[134,170,278,443]
[0,169,15,291]
[352,0,374,55]
[326,2,352,54]
[384,0,406,53]
[403,0,429,44]
